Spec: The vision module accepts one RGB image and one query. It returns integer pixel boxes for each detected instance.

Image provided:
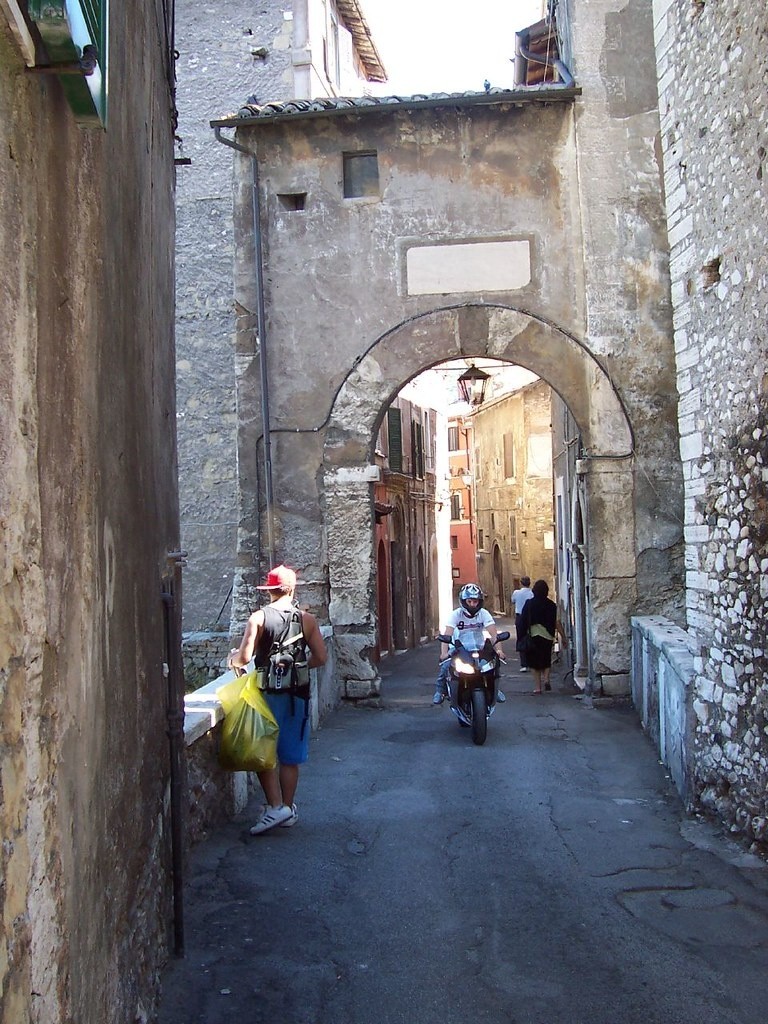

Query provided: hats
[256,565,296,590]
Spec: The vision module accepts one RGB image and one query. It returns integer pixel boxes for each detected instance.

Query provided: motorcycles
[436,632,511,746]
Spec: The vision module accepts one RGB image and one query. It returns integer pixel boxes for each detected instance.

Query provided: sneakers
[496,690,505,703]
[249,804,294,835]
[433,690,448,704]
[278,802,298,826]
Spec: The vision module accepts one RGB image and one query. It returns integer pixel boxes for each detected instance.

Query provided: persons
[511,576,535,672]
[227,564,327,836]
[516,579,559,694]
[432,583,507,705]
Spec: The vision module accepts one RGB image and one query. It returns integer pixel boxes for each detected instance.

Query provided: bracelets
[229,653,238,668]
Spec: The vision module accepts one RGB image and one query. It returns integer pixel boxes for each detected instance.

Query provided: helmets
[459,583,484,618]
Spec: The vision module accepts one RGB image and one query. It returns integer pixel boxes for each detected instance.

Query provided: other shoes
[532,689,542,695]
[519,667,527,672]
[544,680,551,691]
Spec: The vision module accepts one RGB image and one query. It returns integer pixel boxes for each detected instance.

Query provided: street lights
[461,421,473,544]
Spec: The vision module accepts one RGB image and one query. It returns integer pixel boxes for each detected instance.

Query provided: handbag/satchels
[515,631,531,651]
[215,669,278,773]
